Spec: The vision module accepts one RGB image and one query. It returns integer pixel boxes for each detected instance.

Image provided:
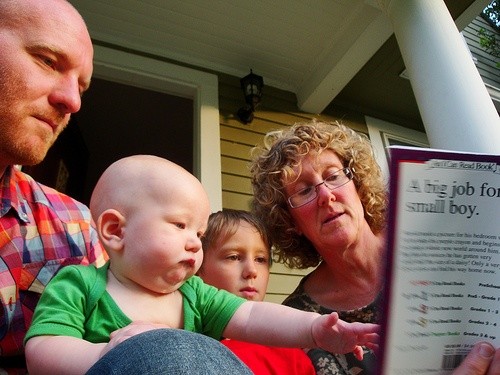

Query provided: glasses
[280,167,356,209]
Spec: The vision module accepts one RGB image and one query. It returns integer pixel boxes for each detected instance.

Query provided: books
[382,145,500,375]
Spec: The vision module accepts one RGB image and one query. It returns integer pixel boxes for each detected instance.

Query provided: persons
[247,120,493,375]
[24,154,381,375]
[0,1,109,374]
[194,208,317,374]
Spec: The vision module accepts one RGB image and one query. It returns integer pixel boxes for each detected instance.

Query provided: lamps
[236,67,265,125]
